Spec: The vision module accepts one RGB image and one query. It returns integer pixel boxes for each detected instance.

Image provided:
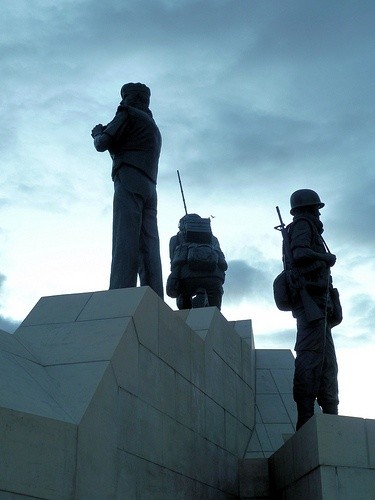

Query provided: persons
[273,189,343,431]
[91,83,164,300]
[169,214,228,312]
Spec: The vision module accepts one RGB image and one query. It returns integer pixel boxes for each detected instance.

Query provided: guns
[274,206,325,326]
